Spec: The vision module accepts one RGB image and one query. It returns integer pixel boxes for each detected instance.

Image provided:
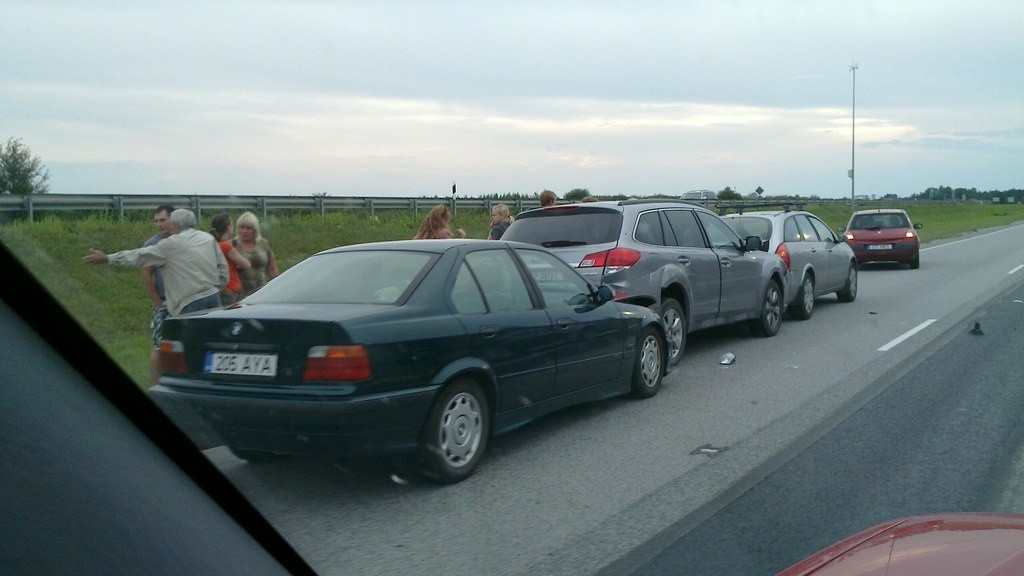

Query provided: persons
[581,197,598,204]
[540,191,557,208]
[487,204,515,240]
[411,204,466,240]
[81,205,279,386]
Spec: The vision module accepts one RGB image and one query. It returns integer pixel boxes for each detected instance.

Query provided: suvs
[679,190,716,205]
[499,198,790,369]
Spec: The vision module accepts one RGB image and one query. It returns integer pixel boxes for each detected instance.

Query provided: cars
[150,239,672,485]
[838,209,923,270]
[718,210,858,320]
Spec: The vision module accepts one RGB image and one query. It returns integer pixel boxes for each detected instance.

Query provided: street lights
[845,65,860,209]
